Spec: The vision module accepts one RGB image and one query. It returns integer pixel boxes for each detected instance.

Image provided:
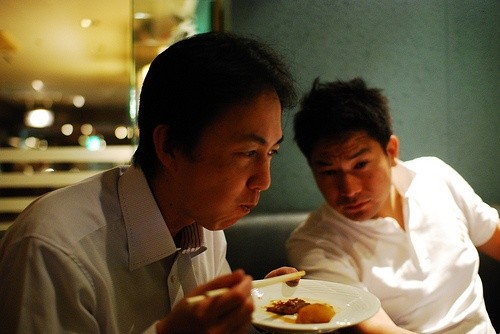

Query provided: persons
[0,32,299,334]
[285,75,500,334]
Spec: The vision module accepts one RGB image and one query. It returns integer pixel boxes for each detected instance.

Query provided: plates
[251,279,381,334]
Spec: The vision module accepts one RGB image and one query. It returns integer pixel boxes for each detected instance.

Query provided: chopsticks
[185,270,305,304]
